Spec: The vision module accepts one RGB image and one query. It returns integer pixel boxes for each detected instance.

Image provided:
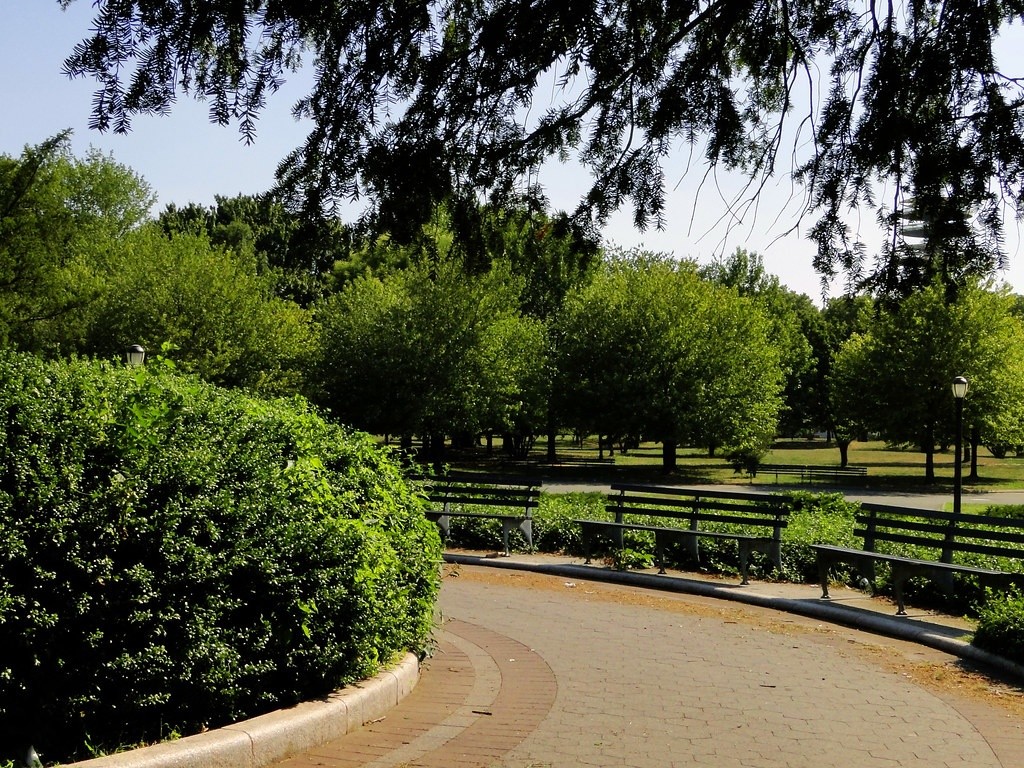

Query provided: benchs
[501,456,616,472]
[746,463,806,483]
[575,484,795,585]
[808,502,1024,615]
[806,465,868,490]
[424,475,544,554]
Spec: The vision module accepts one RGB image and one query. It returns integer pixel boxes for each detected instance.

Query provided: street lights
[951,375,968,529]
